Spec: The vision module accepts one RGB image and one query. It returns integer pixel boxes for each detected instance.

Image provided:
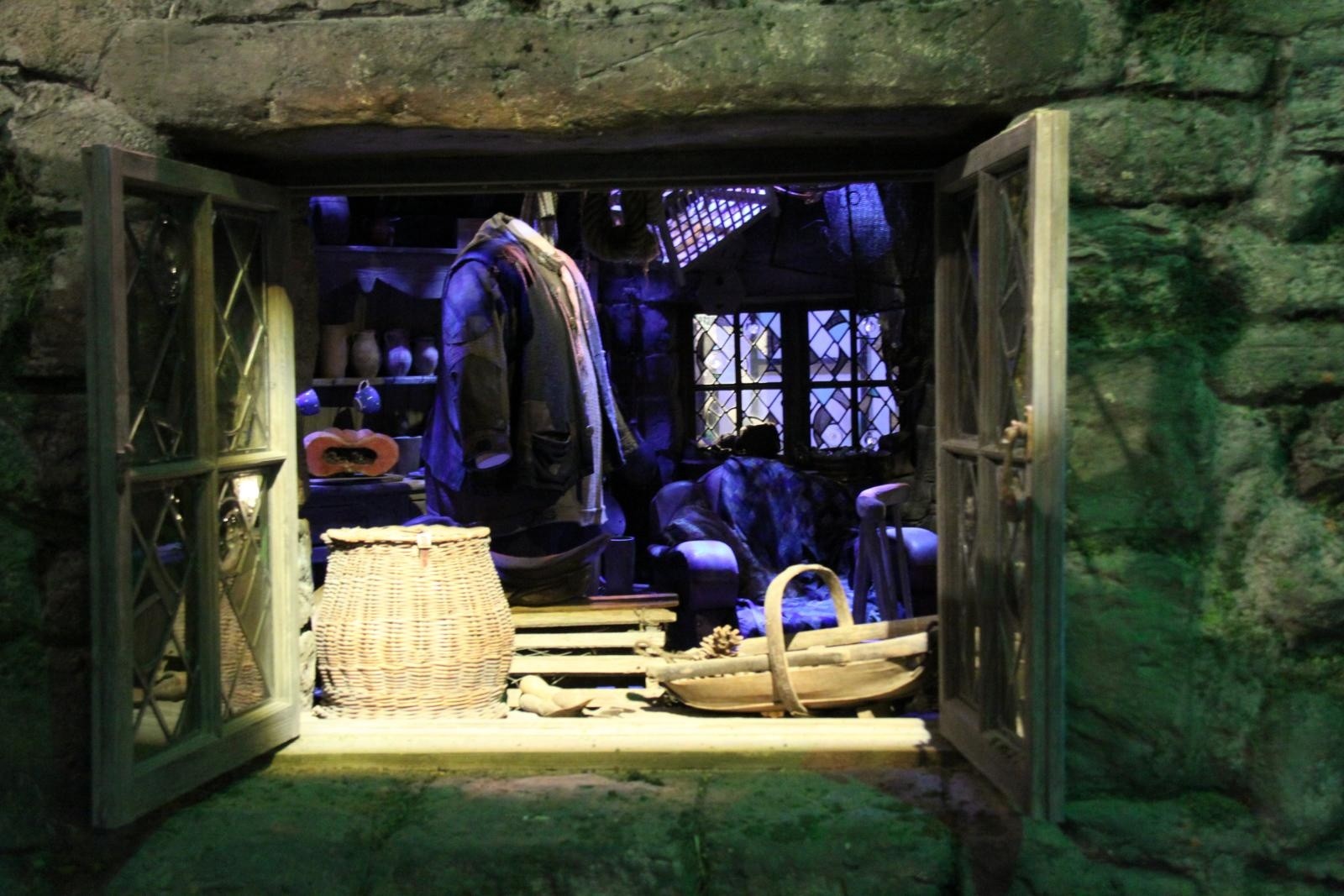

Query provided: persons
[422,215,638,571]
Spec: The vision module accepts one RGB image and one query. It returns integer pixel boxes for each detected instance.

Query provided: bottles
[321,317,440,382]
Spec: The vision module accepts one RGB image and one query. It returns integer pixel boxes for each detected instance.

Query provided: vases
[321,317,442,383]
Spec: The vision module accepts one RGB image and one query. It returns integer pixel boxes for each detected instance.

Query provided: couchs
[646,459,940,644]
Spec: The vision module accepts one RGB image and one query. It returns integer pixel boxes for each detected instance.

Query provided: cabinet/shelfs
[298,212,472,519]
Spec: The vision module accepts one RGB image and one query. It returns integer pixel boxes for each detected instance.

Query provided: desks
[499,581,683,699]
